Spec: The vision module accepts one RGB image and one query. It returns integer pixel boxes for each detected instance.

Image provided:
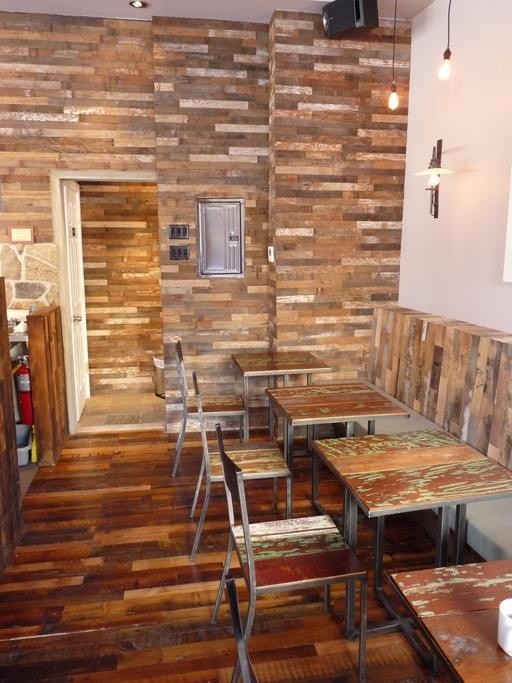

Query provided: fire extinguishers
[16,355,33,430]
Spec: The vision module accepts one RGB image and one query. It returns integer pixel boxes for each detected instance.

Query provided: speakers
[323,0,378,37]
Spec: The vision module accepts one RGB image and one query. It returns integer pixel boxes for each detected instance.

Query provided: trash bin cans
[153,354,164,397]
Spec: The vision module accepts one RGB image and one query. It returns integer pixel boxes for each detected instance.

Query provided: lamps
[415,138,452,218]
